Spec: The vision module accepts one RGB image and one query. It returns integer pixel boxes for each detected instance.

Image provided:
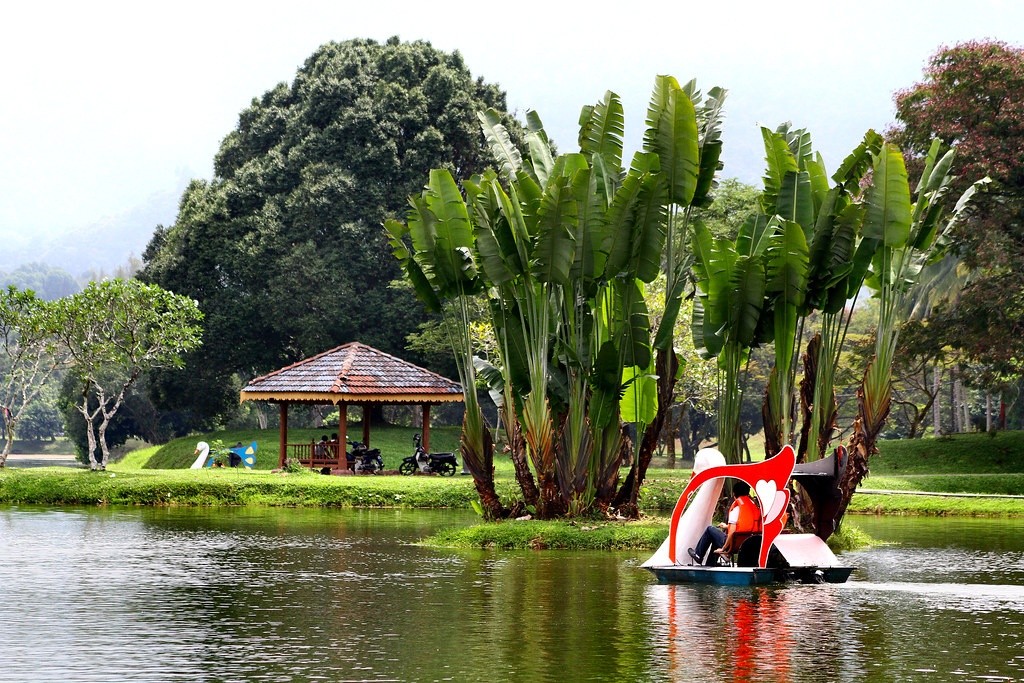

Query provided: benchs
[285,444,358,475]
[714,529,792,568]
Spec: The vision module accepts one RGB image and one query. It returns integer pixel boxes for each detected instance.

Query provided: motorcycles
[399,433,459,476]
[345,435,385,475]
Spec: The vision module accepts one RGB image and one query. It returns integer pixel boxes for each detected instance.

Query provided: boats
[630,446,860,587]
[190,440,258,469]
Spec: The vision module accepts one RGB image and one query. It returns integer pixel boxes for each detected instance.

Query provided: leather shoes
[688,548,703,564]
